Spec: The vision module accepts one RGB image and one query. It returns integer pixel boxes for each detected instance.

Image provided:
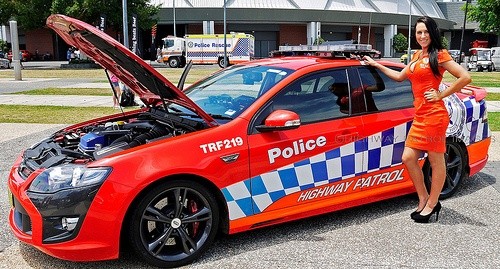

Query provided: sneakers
[131,101,139,106]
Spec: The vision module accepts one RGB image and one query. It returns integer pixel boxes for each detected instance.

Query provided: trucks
[155,31,254,69]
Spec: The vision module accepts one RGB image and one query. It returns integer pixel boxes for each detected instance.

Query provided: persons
[329,61,385,115]
[150,44,162,62]
[35,50,50,61]
[4,47,25,69]
[108,71,125,109]
[67,47,80,66]
[284,43,311,56]
[363,16,473,223]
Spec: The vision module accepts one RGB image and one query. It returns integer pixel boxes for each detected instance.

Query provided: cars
[8,13,491,267]
[447,49,465,62]
[8,50,31,62]
[401,50,415,62]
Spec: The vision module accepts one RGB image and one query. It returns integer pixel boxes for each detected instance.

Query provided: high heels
[411,200,441,223]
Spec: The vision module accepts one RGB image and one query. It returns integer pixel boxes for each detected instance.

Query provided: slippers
[113,104,122,108]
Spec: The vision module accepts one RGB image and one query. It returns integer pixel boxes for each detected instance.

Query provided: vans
[491,47,500,71]
